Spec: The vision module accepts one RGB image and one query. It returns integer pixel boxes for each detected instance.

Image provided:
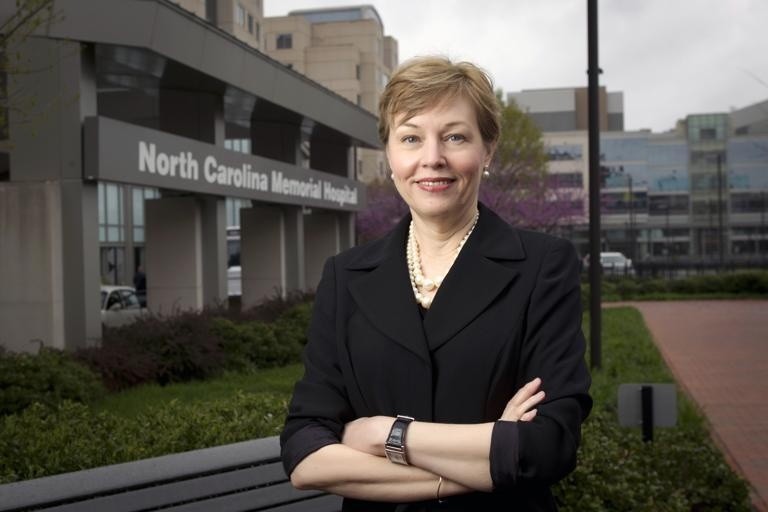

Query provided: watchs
[384,415,414,466]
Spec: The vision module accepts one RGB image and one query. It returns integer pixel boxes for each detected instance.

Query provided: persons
[280,56,594,512]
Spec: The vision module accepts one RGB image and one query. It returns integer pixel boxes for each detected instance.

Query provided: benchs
[1,436,344,510]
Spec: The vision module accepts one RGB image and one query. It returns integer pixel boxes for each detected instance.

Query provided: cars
[99,284,149,331]
[584,250,636,282]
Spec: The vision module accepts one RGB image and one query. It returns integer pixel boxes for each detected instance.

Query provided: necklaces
[405,209,479,309]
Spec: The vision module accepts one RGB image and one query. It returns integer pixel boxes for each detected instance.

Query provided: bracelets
[437,476,444,503]
